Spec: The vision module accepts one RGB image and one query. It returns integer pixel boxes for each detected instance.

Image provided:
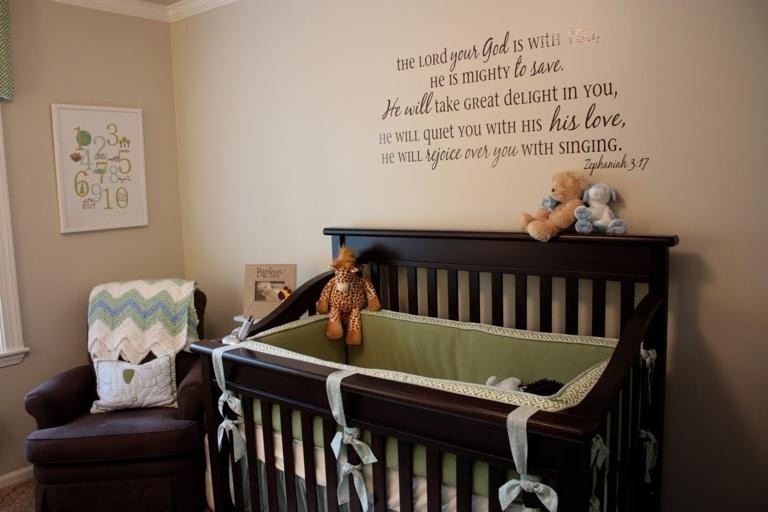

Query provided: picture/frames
[51,104,149,233]
[244,264,297,319]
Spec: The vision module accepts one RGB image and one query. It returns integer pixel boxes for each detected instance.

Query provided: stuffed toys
[574,183,626,234]
[317,245,381,345]
[519,170,587,242]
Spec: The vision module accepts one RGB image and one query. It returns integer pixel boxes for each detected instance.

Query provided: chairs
[25,289,207,512]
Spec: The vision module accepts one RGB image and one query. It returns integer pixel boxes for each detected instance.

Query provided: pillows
[89,351,178,414]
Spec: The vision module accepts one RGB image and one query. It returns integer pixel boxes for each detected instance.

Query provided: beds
[190,228,678,512]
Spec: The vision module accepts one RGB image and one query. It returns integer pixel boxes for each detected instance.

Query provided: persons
[255,281,279,300]
